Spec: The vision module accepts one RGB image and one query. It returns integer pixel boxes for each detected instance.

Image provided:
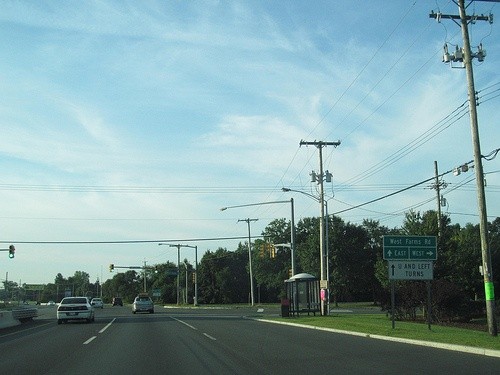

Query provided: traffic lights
[259,244,281,260]
[9,245,14,258]
[193,273,194,284]
[288,269,291,278]
[110,264,114,272]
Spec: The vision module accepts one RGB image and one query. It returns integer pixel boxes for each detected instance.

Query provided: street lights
[219,198,298,314]
[158,243,180,306]
[281,187,330,315]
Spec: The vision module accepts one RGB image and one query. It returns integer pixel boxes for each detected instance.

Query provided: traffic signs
[384,236,437,280]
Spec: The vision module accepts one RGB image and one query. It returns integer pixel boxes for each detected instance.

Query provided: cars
[133,296,154,314]
[112,297,123,307]
[57,297,103,325]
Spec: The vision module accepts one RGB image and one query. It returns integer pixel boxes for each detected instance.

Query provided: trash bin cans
[281,300,290,316]
